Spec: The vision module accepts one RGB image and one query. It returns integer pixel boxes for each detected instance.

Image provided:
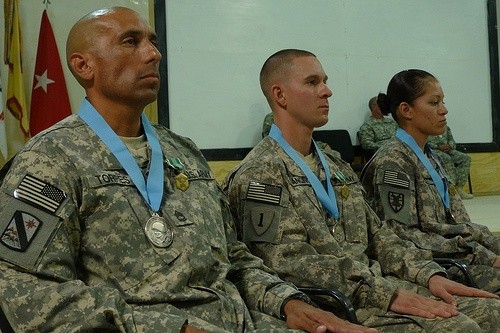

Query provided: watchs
[280,291,311,320]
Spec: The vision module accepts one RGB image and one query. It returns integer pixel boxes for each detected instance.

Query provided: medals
[145,210,174,247]
[332,220,345,242]
[446,205,458,224]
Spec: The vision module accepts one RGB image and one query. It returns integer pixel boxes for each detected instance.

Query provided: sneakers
[458,189,474,199]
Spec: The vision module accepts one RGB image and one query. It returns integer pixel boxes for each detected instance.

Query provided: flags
[0,0,30,161]
[28,9,72,139]
[3,0,28,160]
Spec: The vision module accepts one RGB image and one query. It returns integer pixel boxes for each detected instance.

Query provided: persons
[262,112,274,138]
[0,4,378,333]
[360,96,398,162]
[424,123,474,198]
[359,67,500,298]
[215,47,500,333]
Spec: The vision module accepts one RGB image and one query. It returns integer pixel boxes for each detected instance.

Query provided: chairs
[298,129,477,324]
